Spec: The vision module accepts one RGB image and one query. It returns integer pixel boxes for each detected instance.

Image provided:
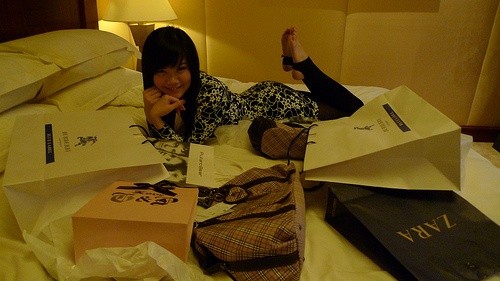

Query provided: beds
[1,0,499,281]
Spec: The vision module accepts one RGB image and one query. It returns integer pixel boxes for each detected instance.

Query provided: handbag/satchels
[70,179,198,265]
[324,180,500,281]
[2,109,171,242]
[190,163,306,281]
[302,84,462,192]
[248,115,308,160]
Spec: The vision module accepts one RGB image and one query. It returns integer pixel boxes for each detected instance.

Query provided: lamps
[96,0,179,72]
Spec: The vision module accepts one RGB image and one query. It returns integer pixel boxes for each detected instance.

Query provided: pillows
[0,67,143,173]
[0,29,142,112]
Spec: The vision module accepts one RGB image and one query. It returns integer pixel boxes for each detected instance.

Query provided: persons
[141,26,364,148]
